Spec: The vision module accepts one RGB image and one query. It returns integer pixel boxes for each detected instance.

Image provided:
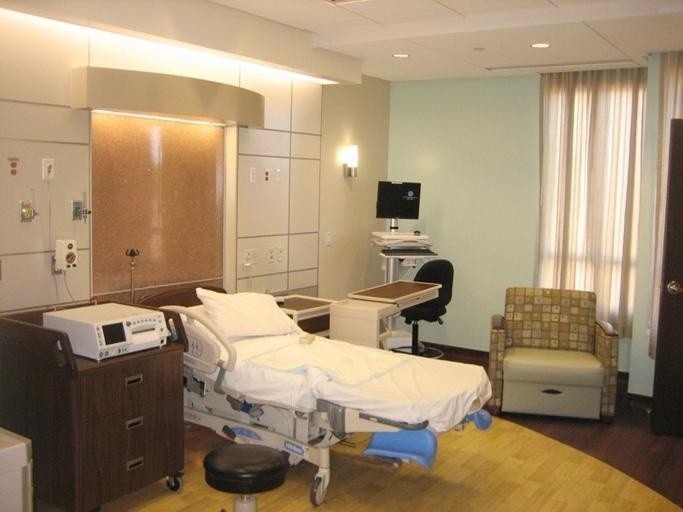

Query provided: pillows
[196,287,304,342]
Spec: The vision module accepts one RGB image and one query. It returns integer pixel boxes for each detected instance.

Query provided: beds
[137,285,493,507]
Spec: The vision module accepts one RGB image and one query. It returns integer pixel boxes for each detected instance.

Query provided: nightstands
[276,294,333,334]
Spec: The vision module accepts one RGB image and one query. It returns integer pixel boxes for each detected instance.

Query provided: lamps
[345,144,357,177]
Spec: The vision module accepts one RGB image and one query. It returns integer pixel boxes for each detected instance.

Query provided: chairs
[389,259,453,359]
[484,287,620,424]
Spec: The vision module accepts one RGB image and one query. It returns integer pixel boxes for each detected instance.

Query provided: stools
[204,444,287,512]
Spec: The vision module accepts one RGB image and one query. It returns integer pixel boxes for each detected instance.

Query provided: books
[370,232,432,249]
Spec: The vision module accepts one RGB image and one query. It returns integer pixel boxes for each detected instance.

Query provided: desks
[329,279,444,348]
